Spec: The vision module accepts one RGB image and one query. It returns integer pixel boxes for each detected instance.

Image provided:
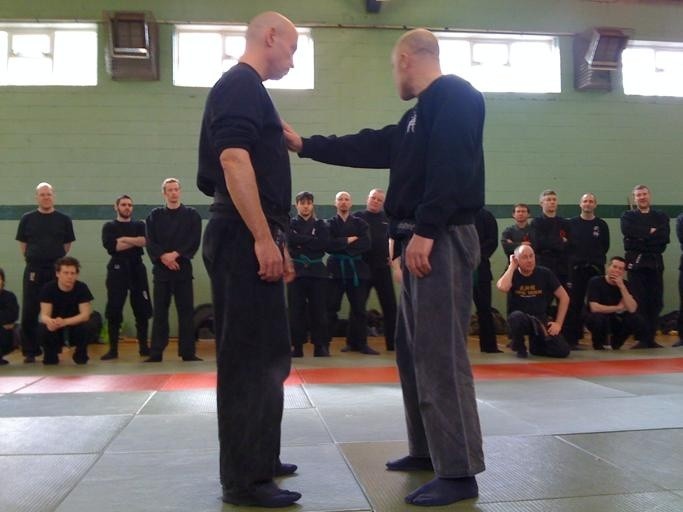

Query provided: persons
[143,178,202,362]
[567,192,610,350]
[502,203,531,348]
[620,184,671,349]
[101,194,153,361]
[473,209,504,353]
[289,191,331,358]
[495,244,571,359]
[324,191,372,354]
[672,212,683,347]
[14,181,77,363]
[34,256,103,365]
[580,256,639,350]
[530,190,571,317]
[196,11,301,507]
[386,227,402,282]
[0,268,21,365]
[276,28,486,506]
[346,188,398,352]
